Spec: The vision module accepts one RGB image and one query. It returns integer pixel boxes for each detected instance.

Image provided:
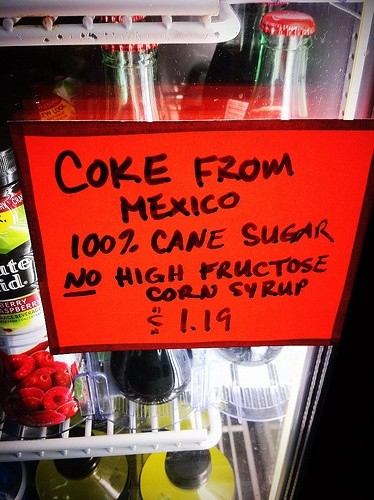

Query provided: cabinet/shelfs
[0,0,374,499]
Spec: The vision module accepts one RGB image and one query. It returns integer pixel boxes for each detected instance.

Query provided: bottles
[34,429,132,500]
[94,11,193,407]
[0,148,89,427]
[217,8,317,365]
[139,446,237,500]
